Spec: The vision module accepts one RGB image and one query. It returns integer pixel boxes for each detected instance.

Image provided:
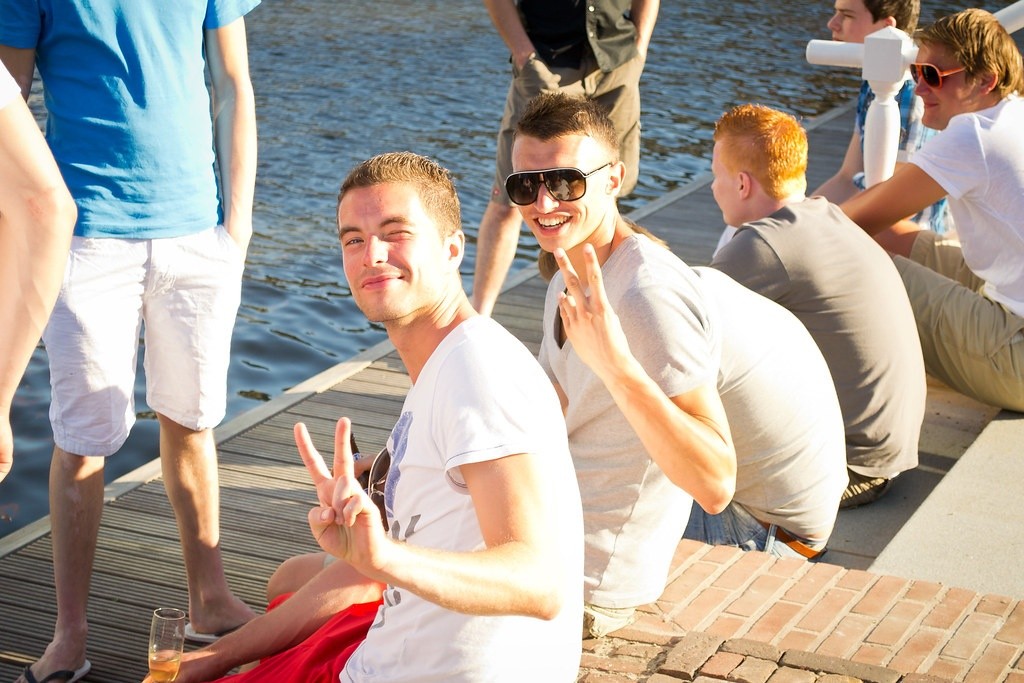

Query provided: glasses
[367,447,392,532]
[504,162,614,207]
[909,63,968,90]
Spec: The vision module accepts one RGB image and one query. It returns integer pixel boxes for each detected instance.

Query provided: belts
[756,520,825,560]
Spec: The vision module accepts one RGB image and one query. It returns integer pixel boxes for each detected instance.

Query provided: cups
[148,608,185,683]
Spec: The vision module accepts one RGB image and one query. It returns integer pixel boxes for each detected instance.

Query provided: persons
[264,99,736,641]
[706,101,929,509]
[0,62,81,487]
[141,149,585,681]
[467,0,659,323]
[0,0,271,683]
[804,0,956,241]
[538,214,848,561]
[828,8,1024,417]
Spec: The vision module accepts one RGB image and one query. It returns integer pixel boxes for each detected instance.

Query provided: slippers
[185,623,246,643]
[23,659,91,682]
